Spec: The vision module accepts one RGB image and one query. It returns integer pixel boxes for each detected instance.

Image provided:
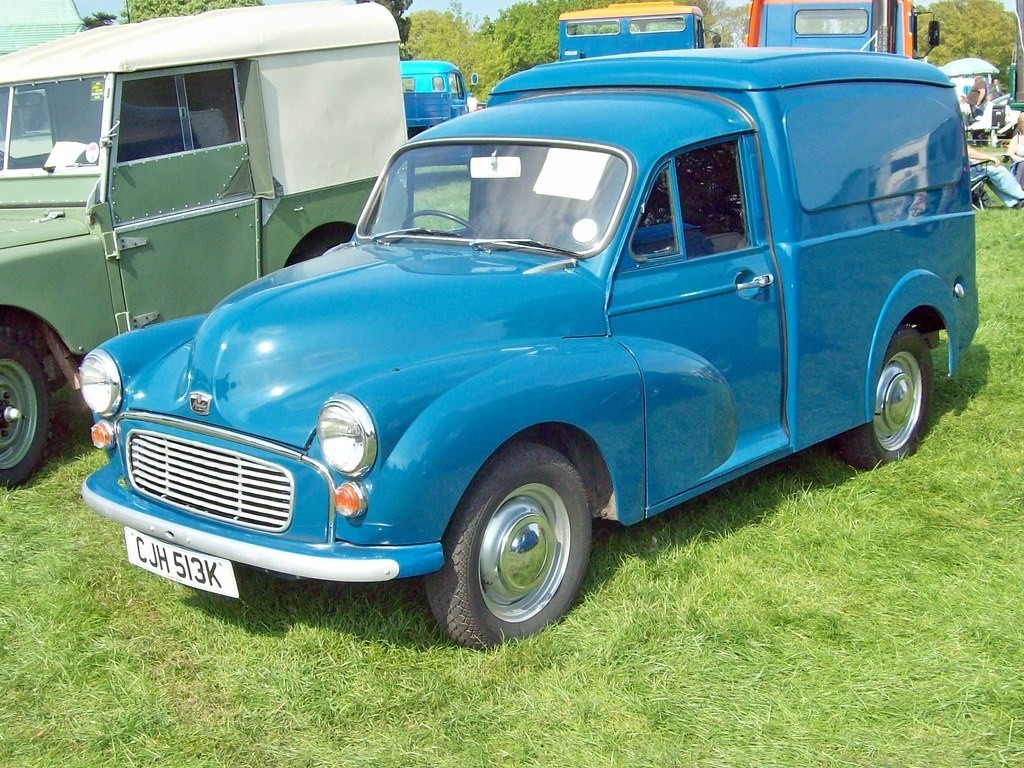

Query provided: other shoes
[1015,199,1024,208]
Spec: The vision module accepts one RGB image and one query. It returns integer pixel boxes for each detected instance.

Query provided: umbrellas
[941,58,999,76]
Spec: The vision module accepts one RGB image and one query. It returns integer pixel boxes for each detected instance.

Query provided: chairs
[968,159,998,211]
[630,224,715,268]
[117,99,203,163]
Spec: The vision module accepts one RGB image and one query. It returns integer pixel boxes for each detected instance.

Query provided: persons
[959,96,971,116]
[967,73,987,140]
[467,92,478,111]
[967,144,1024,209]
[992,78,1002,99]
[1007,112,1024,189]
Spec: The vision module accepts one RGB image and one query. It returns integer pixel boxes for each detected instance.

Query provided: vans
[557,2,722,63]
[0,2,407,492]
[78,48,979,654]
[743,0,941,61]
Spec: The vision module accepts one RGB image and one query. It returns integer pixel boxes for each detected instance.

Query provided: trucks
[398,60,479,141]
